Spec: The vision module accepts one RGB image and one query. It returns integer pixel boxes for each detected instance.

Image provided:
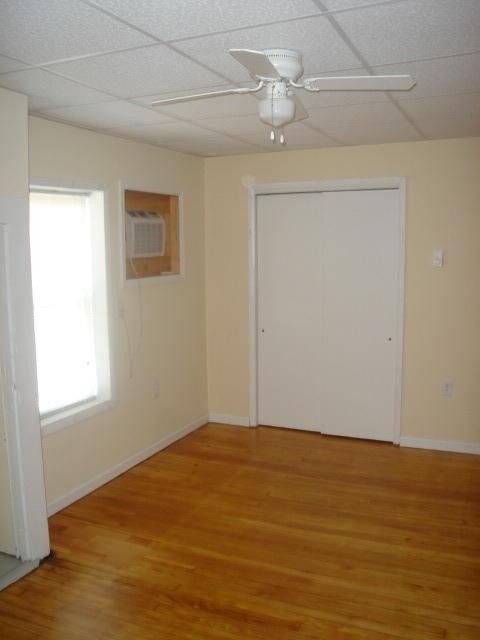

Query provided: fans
[148,46,416,108]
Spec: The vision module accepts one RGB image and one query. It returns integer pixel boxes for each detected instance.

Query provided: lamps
[254,82,302,146]
